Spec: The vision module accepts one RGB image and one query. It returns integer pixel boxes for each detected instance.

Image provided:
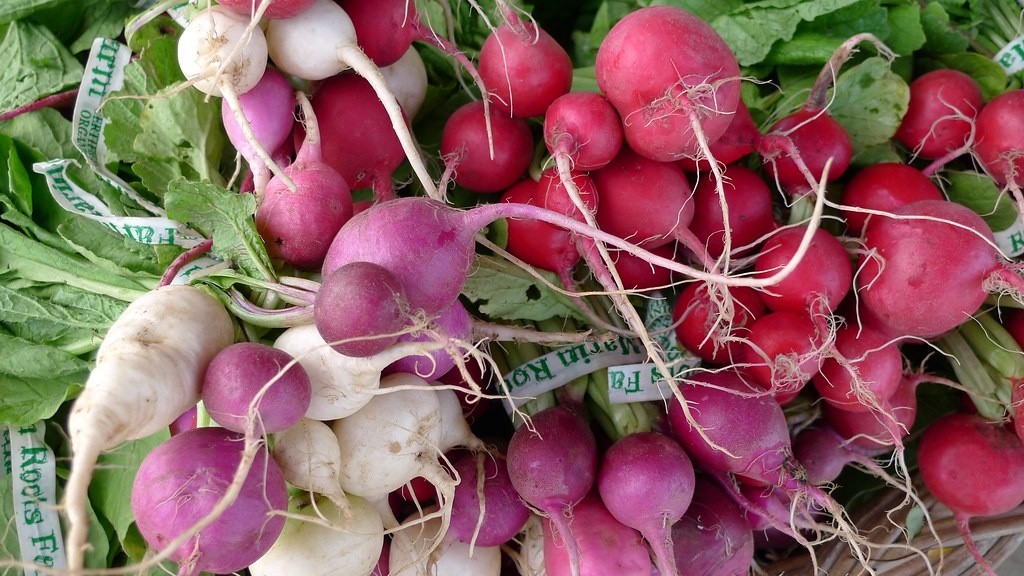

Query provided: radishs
[0,0,545,576]
